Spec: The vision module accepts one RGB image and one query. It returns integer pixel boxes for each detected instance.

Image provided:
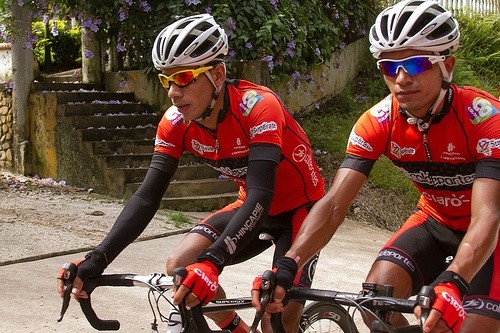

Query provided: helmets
[152,14,230,69]
[368,0,461,57]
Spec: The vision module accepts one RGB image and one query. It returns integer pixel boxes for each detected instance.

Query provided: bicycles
[57,233,456,333]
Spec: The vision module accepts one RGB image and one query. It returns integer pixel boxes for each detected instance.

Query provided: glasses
[158,66,213,89]
[376,55,446,77]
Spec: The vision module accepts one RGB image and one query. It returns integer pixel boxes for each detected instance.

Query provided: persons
[251,0,500,333]
[57,12,325,333]
